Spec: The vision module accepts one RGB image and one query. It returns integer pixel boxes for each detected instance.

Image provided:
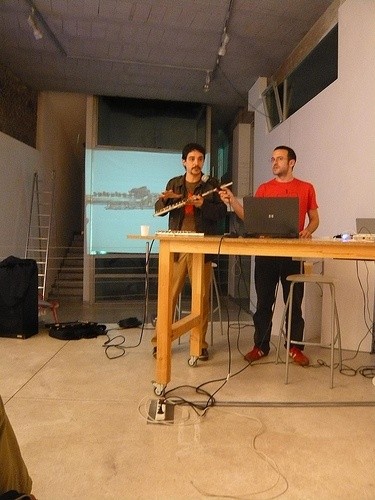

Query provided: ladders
[24,169,57,303]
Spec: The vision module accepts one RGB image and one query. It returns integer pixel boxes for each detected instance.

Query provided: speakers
[0,255,39,340]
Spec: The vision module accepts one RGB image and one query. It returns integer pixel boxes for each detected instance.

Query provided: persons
[151,143,225,360]
[216,145,319,367]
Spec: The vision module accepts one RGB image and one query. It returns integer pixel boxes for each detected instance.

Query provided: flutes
[153,182,233,216]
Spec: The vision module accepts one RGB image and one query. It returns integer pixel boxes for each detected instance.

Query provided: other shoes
[197,348,210,362]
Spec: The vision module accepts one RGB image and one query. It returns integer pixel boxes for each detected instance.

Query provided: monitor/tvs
[356,218,375,234]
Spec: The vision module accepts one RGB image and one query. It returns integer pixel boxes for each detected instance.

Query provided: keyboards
[155,230,205,236]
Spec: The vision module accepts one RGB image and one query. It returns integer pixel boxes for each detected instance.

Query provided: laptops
[243,195,299,240]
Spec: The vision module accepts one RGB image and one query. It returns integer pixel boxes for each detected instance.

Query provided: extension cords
[156,398,167,420]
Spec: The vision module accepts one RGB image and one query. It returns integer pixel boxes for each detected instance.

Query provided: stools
[276,256,343,390]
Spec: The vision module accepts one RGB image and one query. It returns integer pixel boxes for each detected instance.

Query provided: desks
[127,234,375,396]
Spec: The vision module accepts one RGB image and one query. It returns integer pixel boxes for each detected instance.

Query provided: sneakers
[243,347,265,361]
[289,347,310,366]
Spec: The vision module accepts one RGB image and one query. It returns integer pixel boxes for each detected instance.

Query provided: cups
[141,225,150,236]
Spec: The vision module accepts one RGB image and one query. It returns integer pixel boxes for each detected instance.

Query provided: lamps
[26,7,43,40]
[201,69,212,93]
[217,28,230,56]
